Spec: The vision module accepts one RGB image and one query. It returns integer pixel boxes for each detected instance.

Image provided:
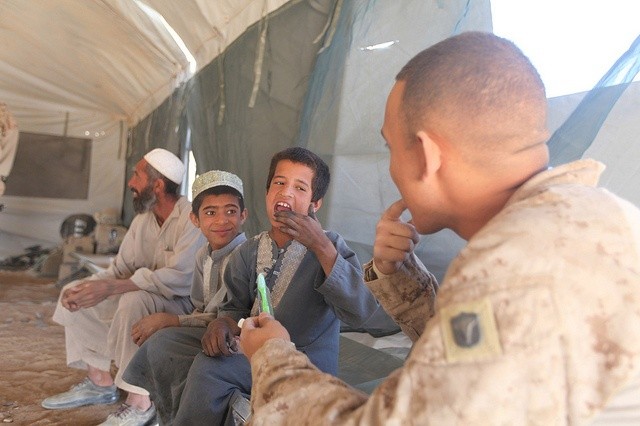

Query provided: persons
[120,147,381,425]
[40,147,209,426]
[131,168,248,347]
[237,31,640,425]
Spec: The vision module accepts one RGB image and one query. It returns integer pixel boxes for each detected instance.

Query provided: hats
[143,148,187,186]
[191,170,244,204]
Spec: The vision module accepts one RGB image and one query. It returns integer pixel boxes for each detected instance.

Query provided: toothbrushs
[256,272,270,312]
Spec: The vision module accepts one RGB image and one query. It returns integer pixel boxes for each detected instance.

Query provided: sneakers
[40,376,122,410]
[96,400,156,426]
